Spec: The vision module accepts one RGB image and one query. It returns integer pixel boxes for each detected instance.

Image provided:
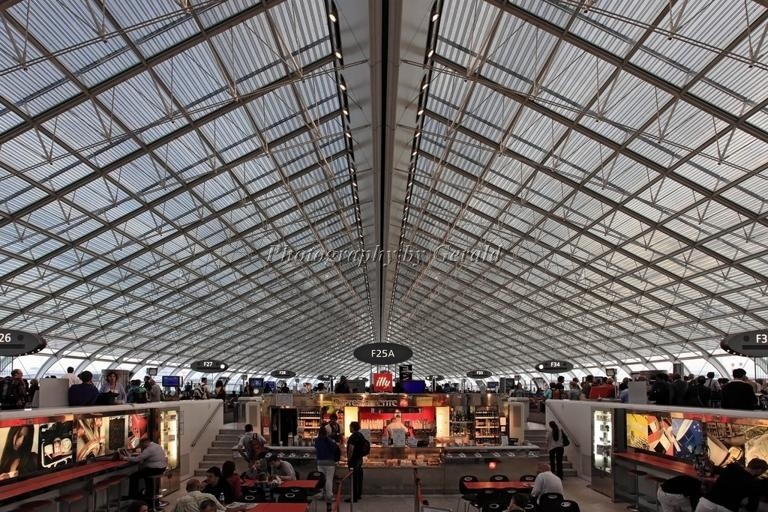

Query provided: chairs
[457,475,580,512]
[239,470,330,512]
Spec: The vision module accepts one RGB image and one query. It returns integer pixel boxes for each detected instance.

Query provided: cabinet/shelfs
[296,405,501,446]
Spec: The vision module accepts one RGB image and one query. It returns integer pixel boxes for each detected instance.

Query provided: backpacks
[193,383,206,398]
[550,387,561,399]
[247,432,265,460]
[356,431,370,457]
[1,377,20,406]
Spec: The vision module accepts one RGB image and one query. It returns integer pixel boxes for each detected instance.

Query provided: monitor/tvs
[509,438,517,446]
[417,439,429,447]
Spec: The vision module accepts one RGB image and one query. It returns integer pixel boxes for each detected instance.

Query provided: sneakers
[328,497,336,502]
[344,497,358,503]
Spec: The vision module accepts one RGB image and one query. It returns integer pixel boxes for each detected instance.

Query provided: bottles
[298,410,320,438]
[287,431,293,446]
[219,492,225,507]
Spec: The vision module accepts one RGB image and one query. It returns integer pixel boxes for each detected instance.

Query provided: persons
[1,369,767,512]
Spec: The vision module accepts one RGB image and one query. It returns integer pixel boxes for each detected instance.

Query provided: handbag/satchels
[561,428,570,446]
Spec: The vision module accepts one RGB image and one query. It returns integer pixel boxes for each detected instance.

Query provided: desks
[0,453,143,512]
[611,451,719,485]
[221,503,310,512]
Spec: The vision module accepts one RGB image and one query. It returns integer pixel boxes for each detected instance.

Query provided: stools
[8,472,170,512]
[624,469,704,512]
